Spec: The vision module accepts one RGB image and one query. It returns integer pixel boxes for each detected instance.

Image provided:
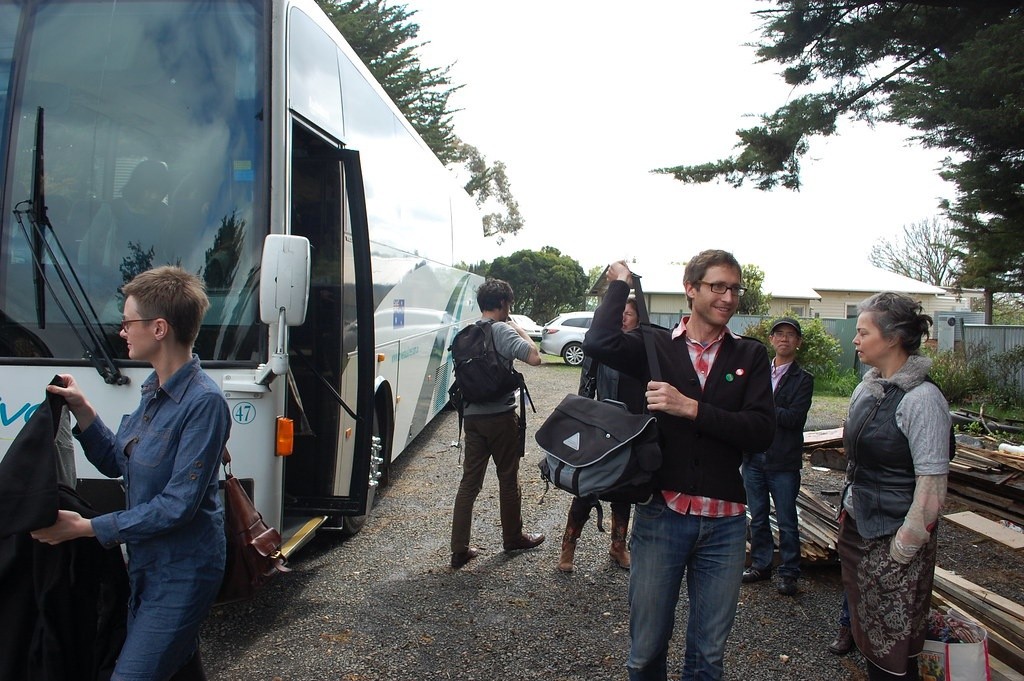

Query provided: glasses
[122,318,174,333]
[773,332,800,339]
[697,280,748,297]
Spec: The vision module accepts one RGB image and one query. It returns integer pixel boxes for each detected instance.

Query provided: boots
[560,498,591,573]
[609,512,631,569]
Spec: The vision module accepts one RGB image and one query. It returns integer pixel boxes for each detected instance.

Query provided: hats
[768,317,801,336]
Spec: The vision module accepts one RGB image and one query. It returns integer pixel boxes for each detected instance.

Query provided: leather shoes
[829,627,854,652]
[452,547,477,565]
[504,532,545,551]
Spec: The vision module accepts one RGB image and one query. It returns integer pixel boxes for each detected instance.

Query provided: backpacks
[448,319,523,408]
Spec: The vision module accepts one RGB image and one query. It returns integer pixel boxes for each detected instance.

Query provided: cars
[505,314,544,342]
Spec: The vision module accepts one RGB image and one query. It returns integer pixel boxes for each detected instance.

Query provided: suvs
[539,311,595,368]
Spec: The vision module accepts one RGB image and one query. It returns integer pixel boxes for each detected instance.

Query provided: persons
[77,160,184,266]
[737,316,814,595]
[451,278,546,567]
[31,265,233,681]
[829,292,956,681]
[558,297,641,572]
[581,249,777,680]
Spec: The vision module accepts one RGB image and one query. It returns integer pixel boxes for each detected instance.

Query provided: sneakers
[779,577,797,594]
[742,569,771,582]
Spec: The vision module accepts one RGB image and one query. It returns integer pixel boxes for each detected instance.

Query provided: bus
[1,1,485,582]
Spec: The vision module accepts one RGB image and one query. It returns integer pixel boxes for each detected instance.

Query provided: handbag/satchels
[219,448,291,591]
[916,608,992,681]
[535,393,661,501]
